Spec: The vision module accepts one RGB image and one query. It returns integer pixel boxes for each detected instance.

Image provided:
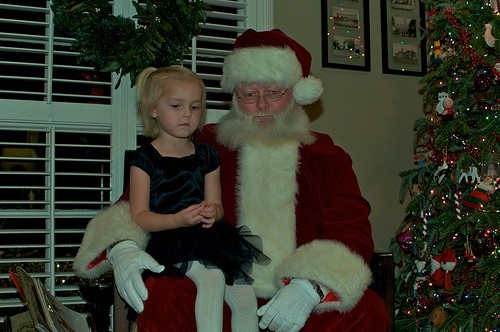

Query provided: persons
[72,29,390,332]
[131,65,271,332]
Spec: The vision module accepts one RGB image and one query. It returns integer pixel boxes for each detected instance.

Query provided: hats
[220,28,324,105]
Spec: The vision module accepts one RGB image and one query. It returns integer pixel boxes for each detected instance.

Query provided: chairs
[113,252,396,332]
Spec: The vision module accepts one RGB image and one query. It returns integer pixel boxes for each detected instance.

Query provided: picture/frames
[379,0,427,77]
[320,0,370,73]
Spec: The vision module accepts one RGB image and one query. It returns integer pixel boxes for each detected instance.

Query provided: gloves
[106,240,166,312]
[257,278,321,332]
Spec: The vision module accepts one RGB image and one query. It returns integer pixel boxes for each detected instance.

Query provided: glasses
[234,88,288,104]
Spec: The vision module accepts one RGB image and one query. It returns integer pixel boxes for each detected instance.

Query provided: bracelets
[309,279,325,301]
